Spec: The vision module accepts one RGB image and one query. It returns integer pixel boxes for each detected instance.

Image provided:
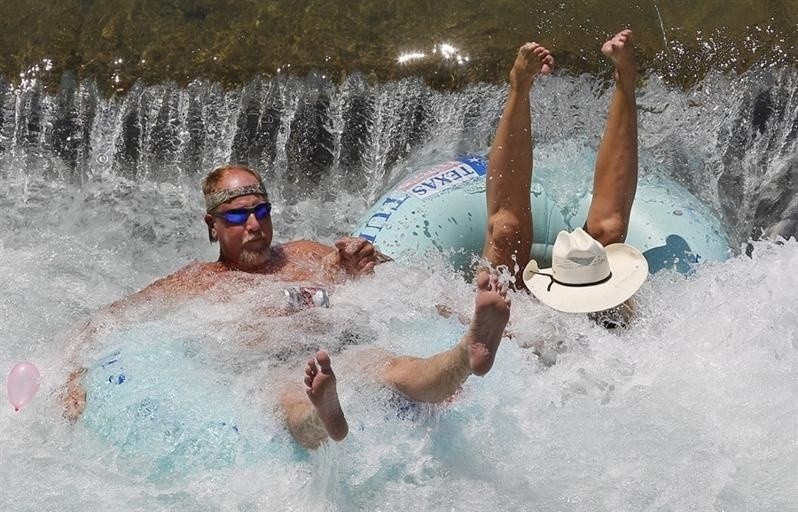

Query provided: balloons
[5,361,41,411]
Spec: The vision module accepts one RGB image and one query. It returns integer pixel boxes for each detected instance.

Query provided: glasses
[208,202,272,226]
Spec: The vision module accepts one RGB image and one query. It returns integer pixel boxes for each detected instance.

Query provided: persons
[59,162,513,455]
[477,26,650,336]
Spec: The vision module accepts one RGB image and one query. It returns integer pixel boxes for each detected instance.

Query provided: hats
[522,227,650,315]
